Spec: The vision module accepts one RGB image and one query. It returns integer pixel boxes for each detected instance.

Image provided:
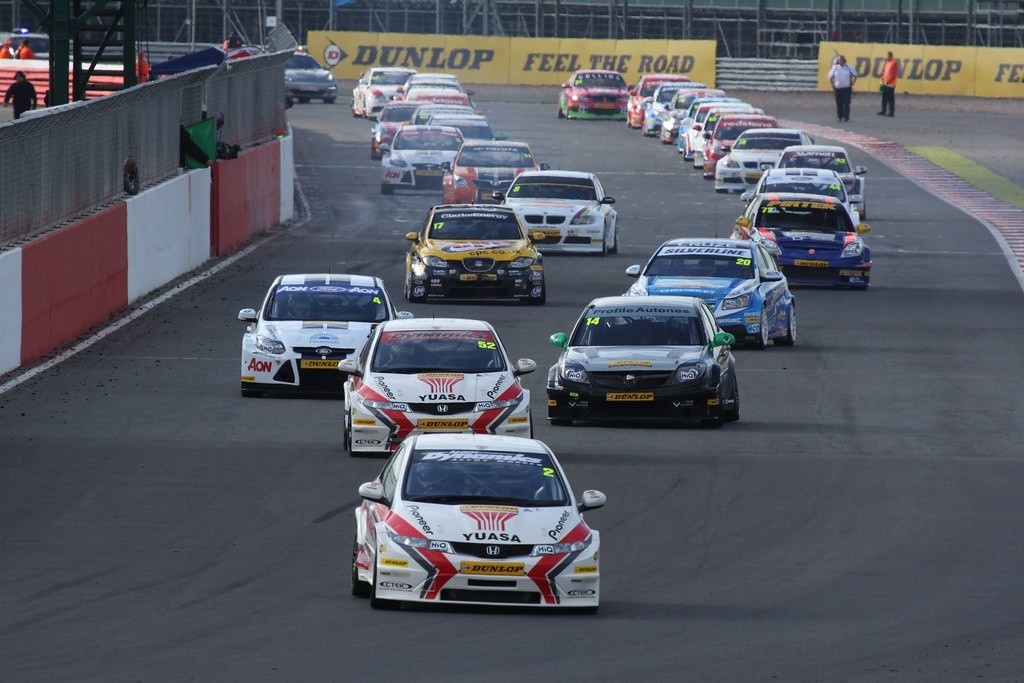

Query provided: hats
[4,38,12,44]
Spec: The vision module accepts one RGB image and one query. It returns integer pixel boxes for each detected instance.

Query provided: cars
[491,169,619,256]
[627,75,815,195]
[351,66,512,194]
[282,51,338,105]
[622,236,799,351]
[238,271,416,399]
[9,33,73,61]
[557,70,634,121]
[403,204,547,307]
[545,296,741,430]
[440,140,550,206]
[338,318,537,459]
[352,433,609,615]
[727,144,872,291]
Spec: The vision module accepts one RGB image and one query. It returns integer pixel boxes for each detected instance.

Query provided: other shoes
[876,111,887,115]
[887,110,894,117]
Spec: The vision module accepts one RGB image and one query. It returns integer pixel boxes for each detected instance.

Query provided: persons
[828,55,857,122]
[664,319,684,344]
[223,37,232,51]
[228,31,241,48]
[0,38,36,59]
[487,221,501,237]
[135,50,150,83]
[877,52,898,117]
[384,342,414,367]
[410,461,454,493]
[123,156,140,195]
[288,294,310,316]
[178,112,213,171]
[4,71,37,120]
[216,112,229,146]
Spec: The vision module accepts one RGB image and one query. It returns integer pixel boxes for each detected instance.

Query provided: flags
[184,117,216,170]
